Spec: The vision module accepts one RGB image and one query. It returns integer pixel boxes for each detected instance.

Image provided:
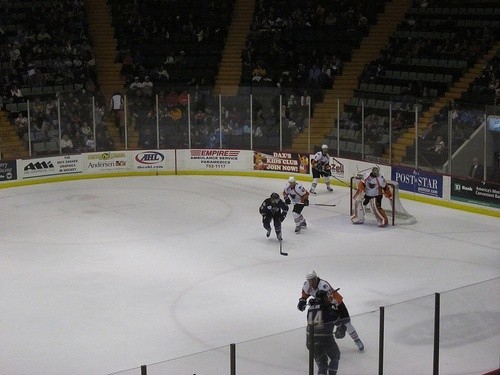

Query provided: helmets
[373,165,380,170]
[321,144,328,149]
[316,290,329,300]
[306,270,316,280]
[289,177,295,183]
[270,193,279,199]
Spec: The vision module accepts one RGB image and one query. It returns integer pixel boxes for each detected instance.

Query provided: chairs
[106,0,500,170]
[0,0,96,154]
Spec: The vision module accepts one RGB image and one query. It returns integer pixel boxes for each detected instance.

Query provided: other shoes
[379,220,388,226]
[353,218,364,224]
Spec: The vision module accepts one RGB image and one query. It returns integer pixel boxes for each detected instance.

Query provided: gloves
[298,297,307,311]
[324,165,330,172]
[284,197,291,204]
[316,163,322,170]
[304,198,309,206]
[335,324,347,338]
[358,180,365,192]
[385,189,393,201]
[277,214,286,222]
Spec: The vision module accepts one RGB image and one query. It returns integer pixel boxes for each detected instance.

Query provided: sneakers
[277,235,282,240]
[327,186,333,191]
[355,339,364,351]
[301,222,306,228]
[309,188,317,196]
[295,225,301,233]
[267,228,271,237]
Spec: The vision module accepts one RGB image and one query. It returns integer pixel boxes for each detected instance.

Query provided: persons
[259,192,290,242]
[309,145,333,196]
[0,0,493,157]
[306,290,347,375]
[297,271,365,353]
[283,177,309,234]
[351,165,393,228]
[414,48,500,196]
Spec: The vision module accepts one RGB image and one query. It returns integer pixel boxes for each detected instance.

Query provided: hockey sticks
[289,202,336,207]
[280,222,288,256]
[322,170,357,190]
[303,287,340,306]
